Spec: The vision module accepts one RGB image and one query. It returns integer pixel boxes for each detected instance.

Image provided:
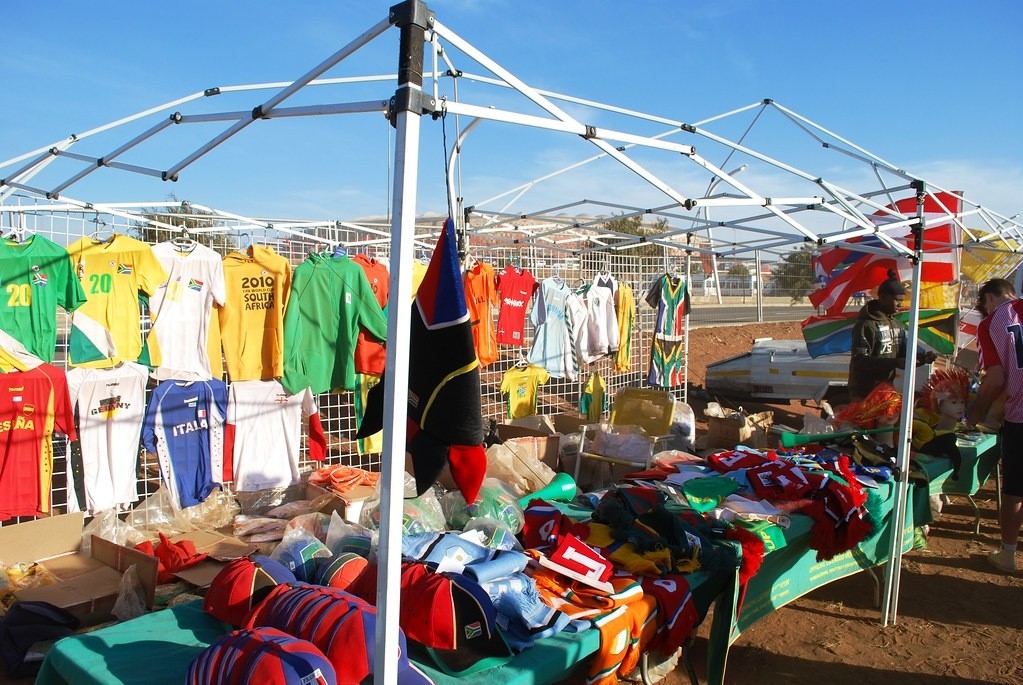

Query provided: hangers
[1,209,678,374]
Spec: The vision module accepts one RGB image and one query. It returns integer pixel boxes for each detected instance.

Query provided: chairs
[574,386,678,485]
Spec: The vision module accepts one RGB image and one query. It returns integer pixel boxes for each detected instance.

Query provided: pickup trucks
[705,262,1023,421]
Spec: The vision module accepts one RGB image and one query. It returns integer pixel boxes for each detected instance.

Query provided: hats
[202,537,515,685]
[877,268,907,295]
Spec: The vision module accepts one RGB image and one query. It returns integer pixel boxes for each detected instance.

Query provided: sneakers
[988,548,1017,573]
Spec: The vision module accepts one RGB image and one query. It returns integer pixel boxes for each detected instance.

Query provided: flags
[809,191,964,318]
[800,307,960,359]
[960,228,1023,289]
[868,280,960,310]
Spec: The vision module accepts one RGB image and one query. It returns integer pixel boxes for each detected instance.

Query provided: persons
[921,370,970,430]
[969,278,1023,573]
[837,382,903,449]
[848,268,937,403]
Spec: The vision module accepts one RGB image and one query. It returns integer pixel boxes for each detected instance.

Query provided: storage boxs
[404,451,461,490]
[496,423,561,469]
[707,409,774,449]
[552,412,597,479]
[1,509,161,630]
[305,480,375,523]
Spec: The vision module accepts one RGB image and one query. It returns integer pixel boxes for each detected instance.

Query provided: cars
[480,253,547,267]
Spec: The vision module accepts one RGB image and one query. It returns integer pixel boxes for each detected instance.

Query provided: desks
[34,432,1002,685]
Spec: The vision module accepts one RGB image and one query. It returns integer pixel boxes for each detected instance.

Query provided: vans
[552,257,582,269]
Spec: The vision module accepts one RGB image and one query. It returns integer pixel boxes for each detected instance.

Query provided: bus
[688,273,764,297]
